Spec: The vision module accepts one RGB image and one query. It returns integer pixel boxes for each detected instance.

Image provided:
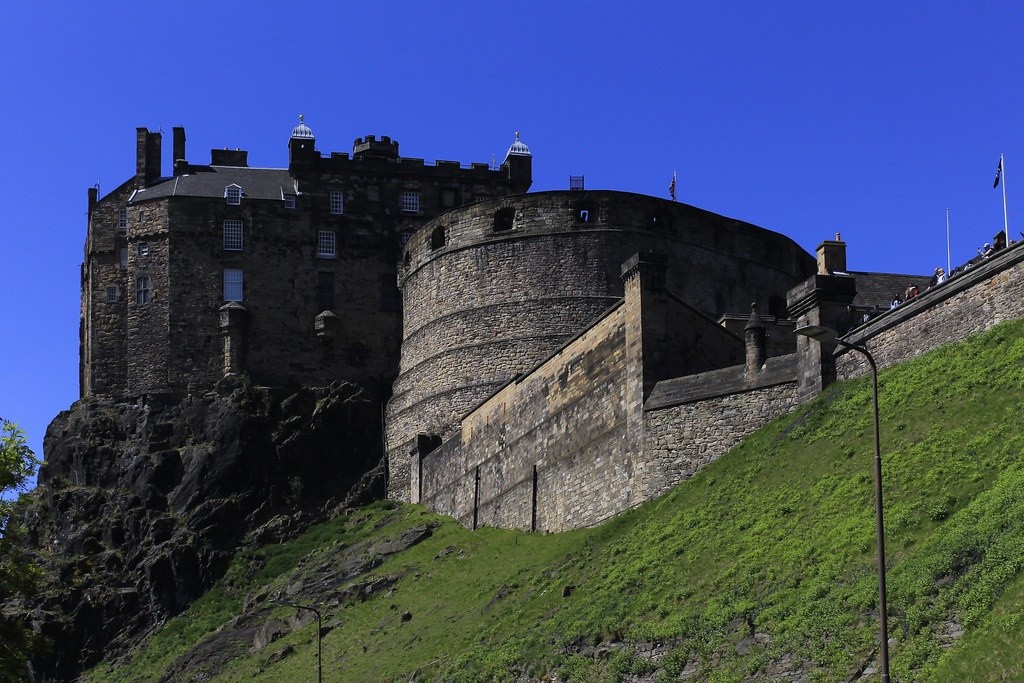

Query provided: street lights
[792,323,891,683]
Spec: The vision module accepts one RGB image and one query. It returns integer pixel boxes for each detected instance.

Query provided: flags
[669,177,676,200]
[994,157,1002,188]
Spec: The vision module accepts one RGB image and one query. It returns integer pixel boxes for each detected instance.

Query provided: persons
[840,305,880,337]
[950,242,1003,278]
[1009,232,1024,246]
[926,267,949,290]
[890,282,921,310]
